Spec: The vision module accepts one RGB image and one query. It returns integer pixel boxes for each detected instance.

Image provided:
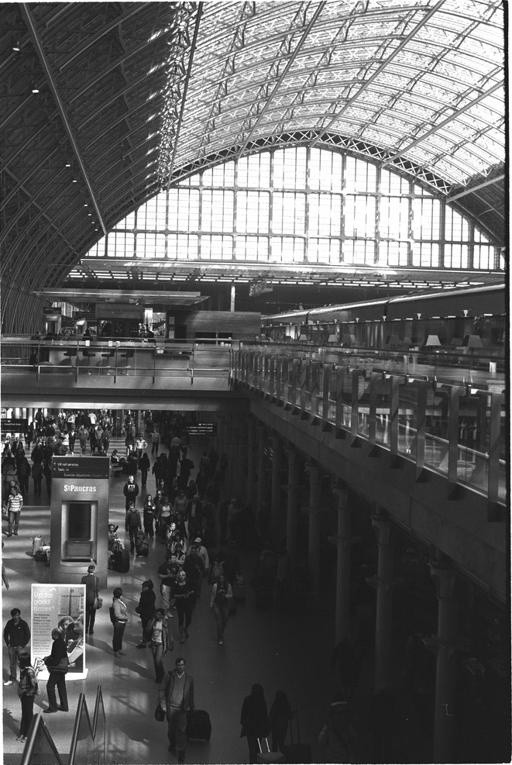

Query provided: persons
[3,608,68,739]
[160,658,194,762]
[242,684,291,762]
[148,608,170,683]
[2,408,242,656]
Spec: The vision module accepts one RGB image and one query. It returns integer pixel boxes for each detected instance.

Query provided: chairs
[82,348,95,375]
[62,348,79,373]
[120,348,137,375]
[101,348,116,376]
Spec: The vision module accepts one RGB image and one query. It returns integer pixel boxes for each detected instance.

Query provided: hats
[194,537,202,542]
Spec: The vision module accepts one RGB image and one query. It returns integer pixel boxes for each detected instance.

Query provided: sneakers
[57,706,68,711]
[44,708,57,712]
[16,734,28,742]
[3,680,13,686]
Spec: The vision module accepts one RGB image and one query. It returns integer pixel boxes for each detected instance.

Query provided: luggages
[256,736,283,764]
[135,530,149,556]
[188,709,211,740]
[284,718,311,764]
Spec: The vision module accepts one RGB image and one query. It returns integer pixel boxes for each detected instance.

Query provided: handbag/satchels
[155,700,165,721]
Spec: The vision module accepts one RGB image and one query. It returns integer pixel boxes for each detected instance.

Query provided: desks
[228,328,508,490]
